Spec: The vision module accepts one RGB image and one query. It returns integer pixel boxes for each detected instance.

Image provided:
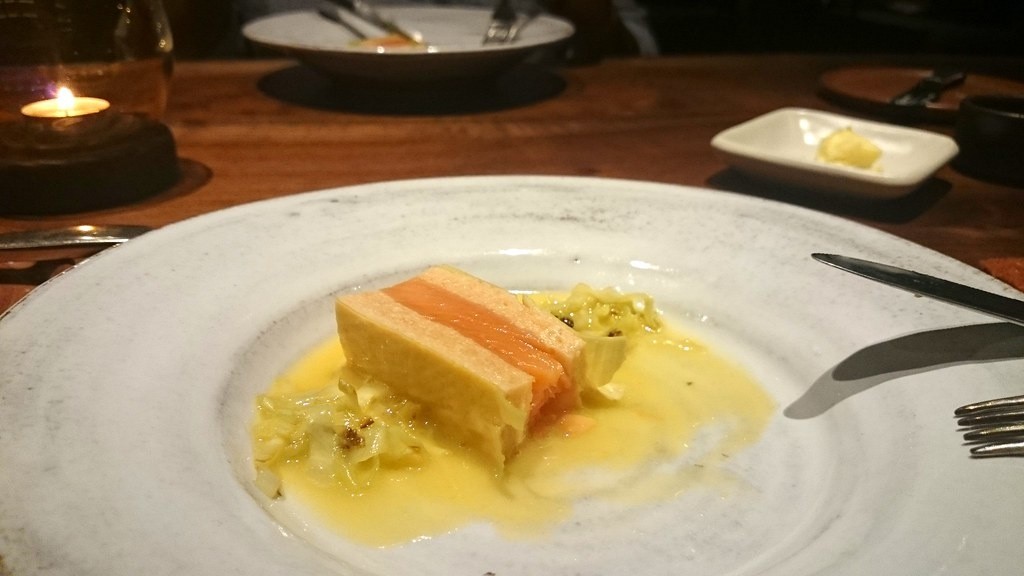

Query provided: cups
[969,94,1024,143]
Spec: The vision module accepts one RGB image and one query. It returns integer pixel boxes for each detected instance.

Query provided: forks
[954,394,1024,454]
[480,0,544,40]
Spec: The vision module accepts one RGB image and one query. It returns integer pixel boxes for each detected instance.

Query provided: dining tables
[0,56,1024,576]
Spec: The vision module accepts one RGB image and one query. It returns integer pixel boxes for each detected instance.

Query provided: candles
[22,98,111,147]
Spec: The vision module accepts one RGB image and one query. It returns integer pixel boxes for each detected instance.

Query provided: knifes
[891,68,966,107]
[812,253,1024,324]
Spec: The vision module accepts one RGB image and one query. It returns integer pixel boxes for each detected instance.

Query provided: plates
[818,66,974,108]
[1,173,1024,576]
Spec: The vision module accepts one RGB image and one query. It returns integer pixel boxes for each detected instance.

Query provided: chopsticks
[310,0,422,41]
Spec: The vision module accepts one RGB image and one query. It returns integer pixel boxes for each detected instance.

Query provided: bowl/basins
[711,106,960,196]
[240,7,576,79]
